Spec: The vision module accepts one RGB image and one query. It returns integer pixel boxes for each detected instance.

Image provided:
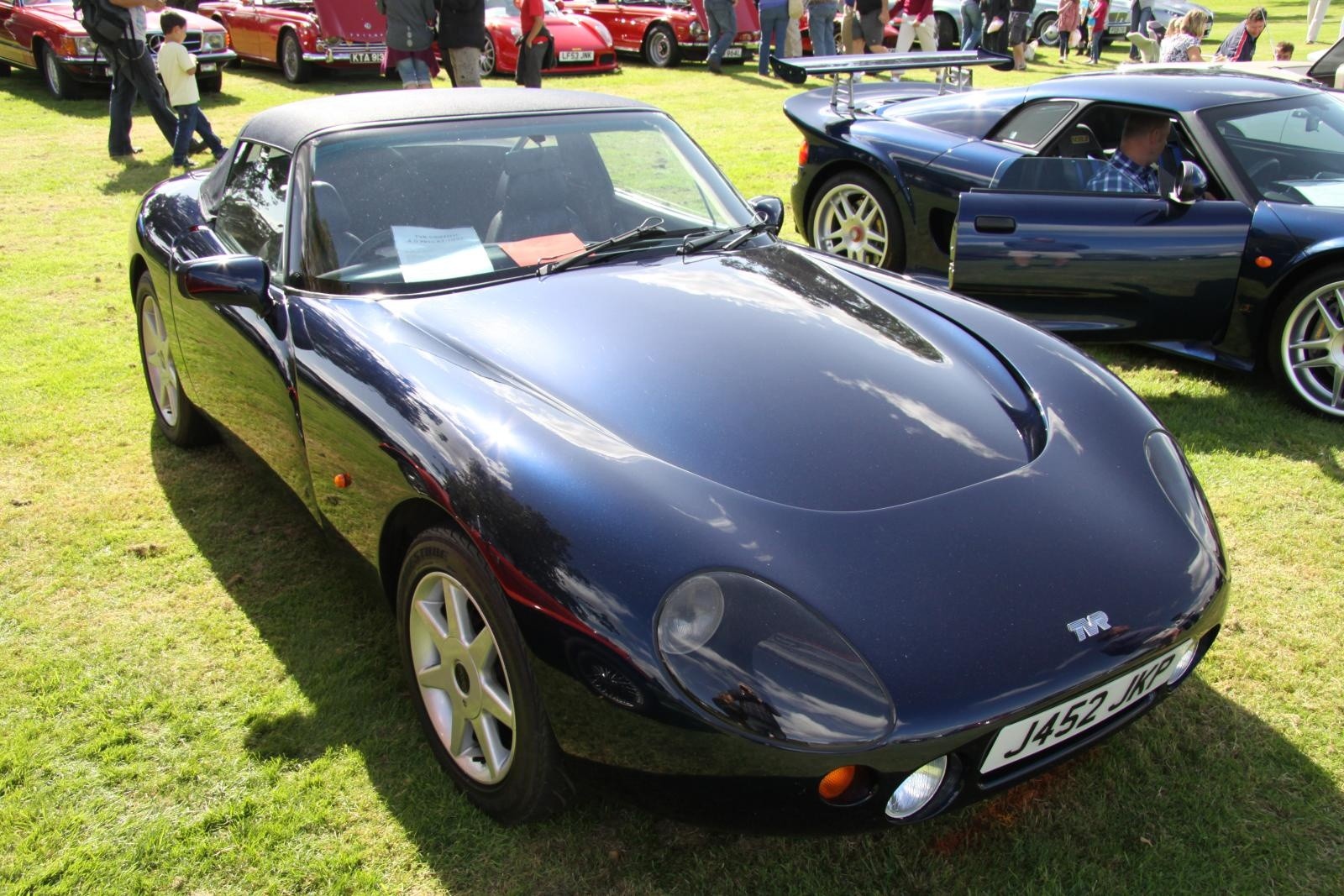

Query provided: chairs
[488,146,596,242]
[1057,123,1110,192]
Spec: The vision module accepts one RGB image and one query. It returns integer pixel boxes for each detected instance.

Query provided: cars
[0,1,1226,106]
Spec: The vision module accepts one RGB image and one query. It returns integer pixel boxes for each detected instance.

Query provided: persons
[84,0,209,159]
[839,0,897,84]
[157,11,228,168]
[1274,41,1294,61]
[754,0,803,79]
[1057,0,1112,66]
[1085,110,1218,201]
[960,0,1036,70]
[888,0,942,84]
[803,0,839,80]
[377,0,438,90]
[703,0,738,73]
[1159,8,1227,64]
[1306,0,1331,44]
[1214,7,1267,62]
[1129,0,1155,58]
[433,0,485,87]
[515,0,549,87]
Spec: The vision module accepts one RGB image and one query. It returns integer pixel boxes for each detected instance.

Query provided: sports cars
[124,78,1236,835]
[762,43,1344,427]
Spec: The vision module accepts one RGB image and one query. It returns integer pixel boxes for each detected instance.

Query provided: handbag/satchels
[81,0,131,47]
[1088,18,1096,26]
[1068,29,1082,48]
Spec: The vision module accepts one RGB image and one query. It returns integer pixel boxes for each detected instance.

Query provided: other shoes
[113,148,144,157]
[212,148,229,161]
[195,139,209,153]
[175,159,196,167]
[705,58,723,73]
[839,77,860,84]
